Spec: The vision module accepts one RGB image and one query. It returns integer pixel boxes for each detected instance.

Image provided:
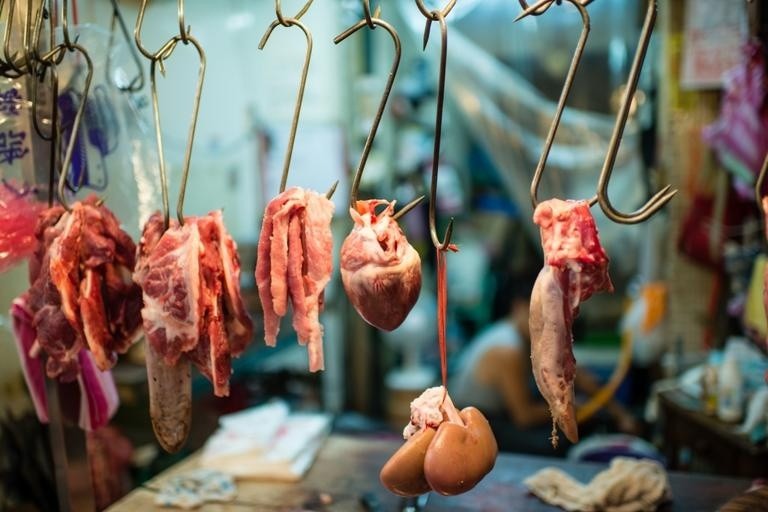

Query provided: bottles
[717,350,743,424]
[701,367,718,417]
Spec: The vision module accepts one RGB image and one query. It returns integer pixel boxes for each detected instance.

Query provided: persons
[443,274,640,462]
